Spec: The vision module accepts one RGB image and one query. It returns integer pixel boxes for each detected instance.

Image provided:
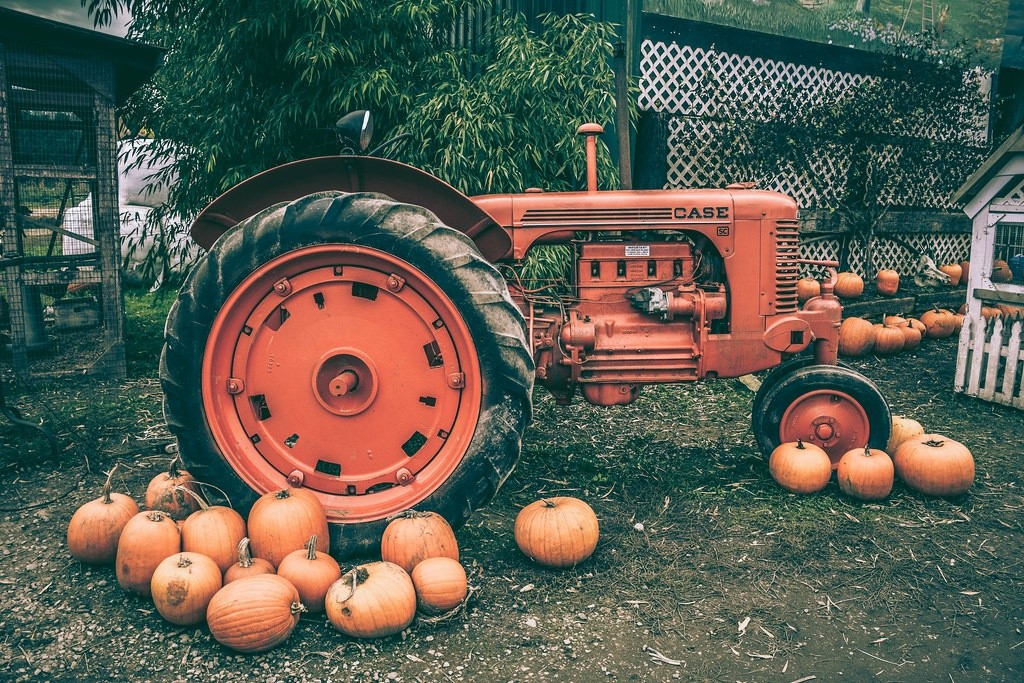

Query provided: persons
[122,125,148,140]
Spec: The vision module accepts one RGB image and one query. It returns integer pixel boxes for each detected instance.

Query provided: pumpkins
[58,237,1021,673]
